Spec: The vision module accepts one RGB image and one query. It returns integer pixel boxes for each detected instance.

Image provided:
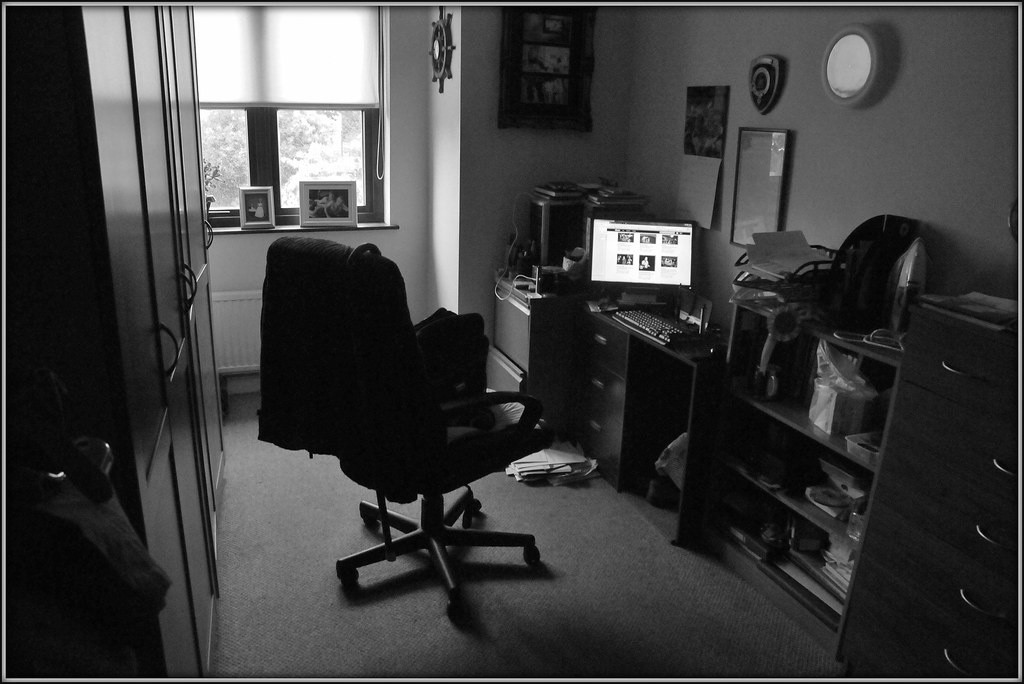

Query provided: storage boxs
[844,431,883,464]
[817,456,870,492]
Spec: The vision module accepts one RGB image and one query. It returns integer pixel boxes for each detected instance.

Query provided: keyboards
[611,310,685,346]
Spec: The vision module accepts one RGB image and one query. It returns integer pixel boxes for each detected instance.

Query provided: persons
[309,190,348,218]
[254,197,265,218]
[618,233,677,269]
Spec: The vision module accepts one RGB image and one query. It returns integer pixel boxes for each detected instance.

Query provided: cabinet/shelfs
[6,5,217,678]
[483,268,582,439]
[834,305,1018,678]
[161,6,226,598]
[569,300,729,546]
[695,297,901,664]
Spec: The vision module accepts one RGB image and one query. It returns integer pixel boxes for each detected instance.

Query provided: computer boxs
[527,196,646,280]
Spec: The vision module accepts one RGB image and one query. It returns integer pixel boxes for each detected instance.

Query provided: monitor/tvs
[585,213,697,311]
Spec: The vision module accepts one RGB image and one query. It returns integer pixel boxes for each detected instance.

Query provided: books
[921,293,1017,331]
[822,536,858,589]
[533,179,648,206]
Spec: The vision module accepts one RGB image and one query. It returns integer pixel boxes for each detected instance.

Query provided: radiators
[212,290,262,374]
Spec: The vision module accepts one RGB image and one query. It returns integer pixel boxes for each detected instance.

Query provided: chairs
[257,236,556,625]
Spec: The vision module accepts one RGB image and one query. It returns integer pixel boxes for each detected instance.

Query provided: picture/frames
[497,6,598,133]
[299,181,358,227]
[239,186,275,229]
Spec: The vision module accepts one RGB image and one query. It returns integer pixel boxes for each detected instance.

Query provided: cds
[809,487,852,507]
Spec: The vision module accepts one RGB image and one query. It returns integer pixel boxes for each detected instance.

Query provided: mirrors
[821,24,884,109]
[728,127,792,249]
